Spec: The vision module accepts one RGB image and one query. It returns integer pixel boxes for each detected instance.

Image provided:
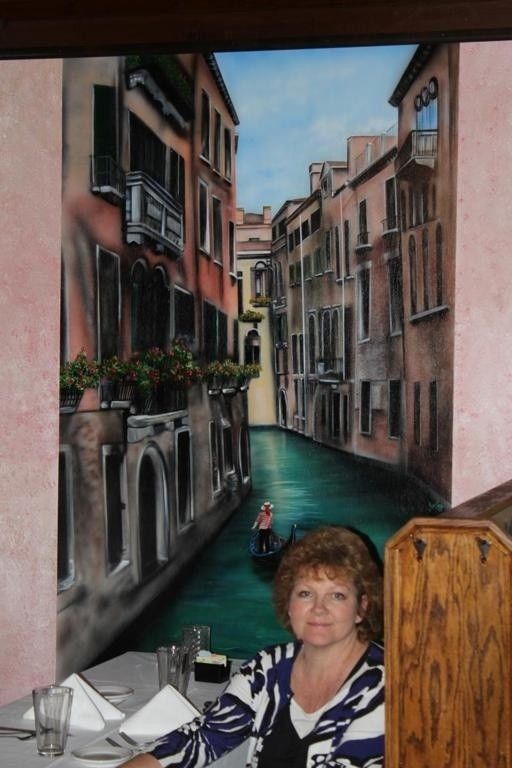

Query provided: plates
[72,745,134,762]
[96,685,135,698]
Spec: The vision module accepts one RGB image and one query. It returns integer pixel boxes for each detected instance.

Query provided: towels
[117,683,209,741]
[21,670,133,733]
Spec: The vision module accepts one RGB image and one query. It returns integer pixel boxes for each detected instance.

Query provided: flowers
[60,340,263,419]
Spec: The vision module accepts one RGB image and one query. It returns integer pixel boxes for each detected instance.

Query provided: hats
[258,501,274,511]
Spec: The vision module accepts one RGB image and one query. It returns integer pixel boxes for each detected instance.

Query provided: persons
[249,502,274,553]
[111,523,386,767]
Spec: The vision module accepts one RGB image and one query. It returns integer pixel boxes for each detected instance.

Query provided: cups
[157,646,189,695]
[181,623,213,670]
[31,685,74,757]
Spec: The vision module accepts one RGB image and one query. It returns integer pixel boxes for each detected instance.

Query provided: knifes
[2,727,74,738]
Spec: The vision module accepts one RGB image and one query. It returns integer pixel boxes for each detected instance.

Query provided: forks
[106,731,169,752]
[1,726,54,741]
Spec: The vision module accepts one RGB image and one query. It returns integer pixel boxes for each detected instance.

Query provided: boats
[248,523,297,562]
